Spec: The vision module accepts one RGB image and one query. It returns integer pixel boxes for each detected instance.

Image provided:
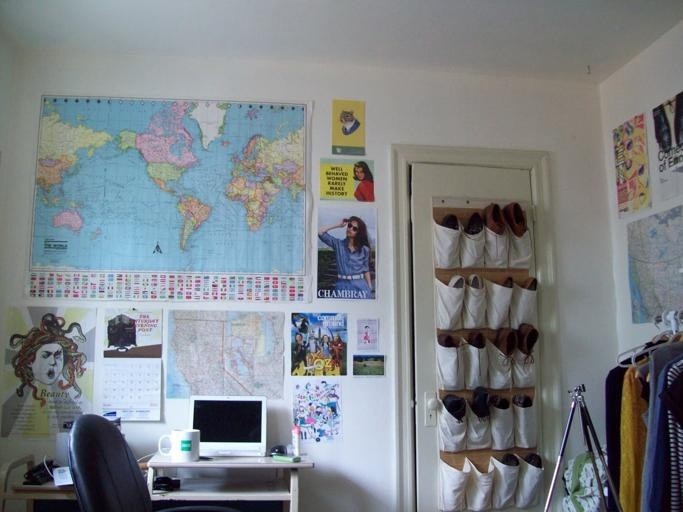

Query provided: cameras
[153,477,180,491]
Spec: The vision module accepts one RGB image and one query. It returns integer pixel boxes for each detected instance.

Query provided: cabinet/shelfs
[1,455,147,510]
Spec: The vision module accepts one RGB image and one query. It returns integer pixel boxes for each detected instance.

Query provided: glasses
[348,223,357,231]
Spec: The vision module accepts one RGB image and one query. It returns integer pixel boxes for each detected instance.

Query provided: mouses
[269,446,284,456]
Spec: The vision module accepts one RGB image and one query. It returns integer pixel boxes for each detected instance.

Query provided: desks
[146,447,314,512]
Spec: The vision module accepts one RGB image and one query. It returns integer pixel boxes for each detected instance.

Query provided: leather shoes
[438,203,538,417]
[501,452,541,468]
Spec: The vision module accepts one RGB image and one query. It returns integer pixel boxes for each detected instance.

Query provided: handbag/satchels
[562,445,609,512]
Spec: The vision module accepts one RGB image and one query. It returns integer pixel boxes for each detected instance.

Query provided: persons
[317,217,373,298]
[352,162,374,201]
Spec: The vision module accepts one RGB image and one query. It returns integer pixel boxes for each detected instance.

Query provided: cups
[159,428,201,463]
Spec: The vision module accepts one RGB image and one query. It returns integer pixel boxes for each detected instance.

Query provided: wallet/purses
[23,461,60,485]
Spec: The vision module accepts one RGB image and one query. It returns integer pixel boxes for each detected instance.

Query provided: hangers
[615,307,682,369]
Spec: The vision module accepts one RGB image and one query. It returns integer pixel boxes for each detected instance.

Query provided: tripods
[543,384,624,512]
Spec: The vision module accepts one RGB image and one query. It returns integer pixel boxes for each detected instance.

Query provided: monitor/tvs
[189,395,267,457]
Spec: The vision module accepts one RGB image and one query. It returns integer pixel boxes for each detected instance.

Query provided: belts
[338,274,364,279]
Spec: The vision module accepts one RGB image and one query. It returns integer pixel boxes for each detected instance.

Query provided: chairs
[67,413,239,512]
